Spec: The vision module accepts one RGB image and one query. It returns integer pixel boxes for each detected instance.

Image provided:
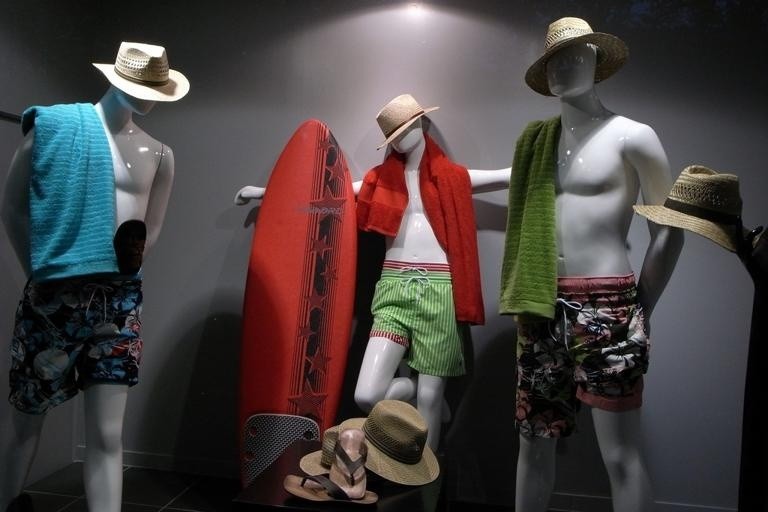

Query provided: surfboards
[236,119,358,512]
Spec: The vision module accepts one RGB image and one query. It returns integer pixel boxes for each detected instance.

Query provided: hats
[298,425,340,478]
[524,17,632,97]
[631,164,760,255]
[338,397,442,486]
[91,40,191,103]
[376,93,440,151]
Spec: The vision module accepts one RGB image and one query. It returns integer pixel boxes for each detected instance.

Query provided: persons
[506,17,686,510]
[2,41,191,512]
[234,91,517,512]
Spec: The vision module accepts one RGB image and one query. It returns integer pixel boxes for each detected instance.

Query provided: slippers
[329,426,370,500]
[282,472,379,506]
